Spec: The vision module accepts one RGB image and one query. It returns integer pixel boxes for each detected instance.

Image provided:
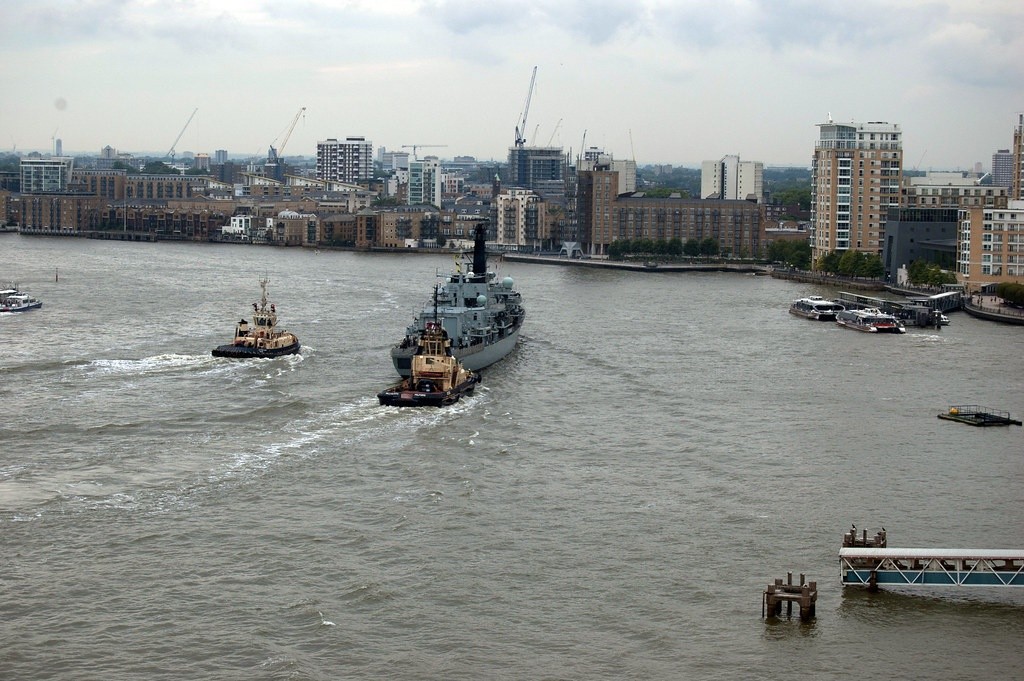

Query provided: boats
[211,268,301,361]
[390,221,525,380]
[0,285,42,312]
[376,285,483,407]
[835,307,906,333]
[936,311,951,325]
[790,294,845,321]
